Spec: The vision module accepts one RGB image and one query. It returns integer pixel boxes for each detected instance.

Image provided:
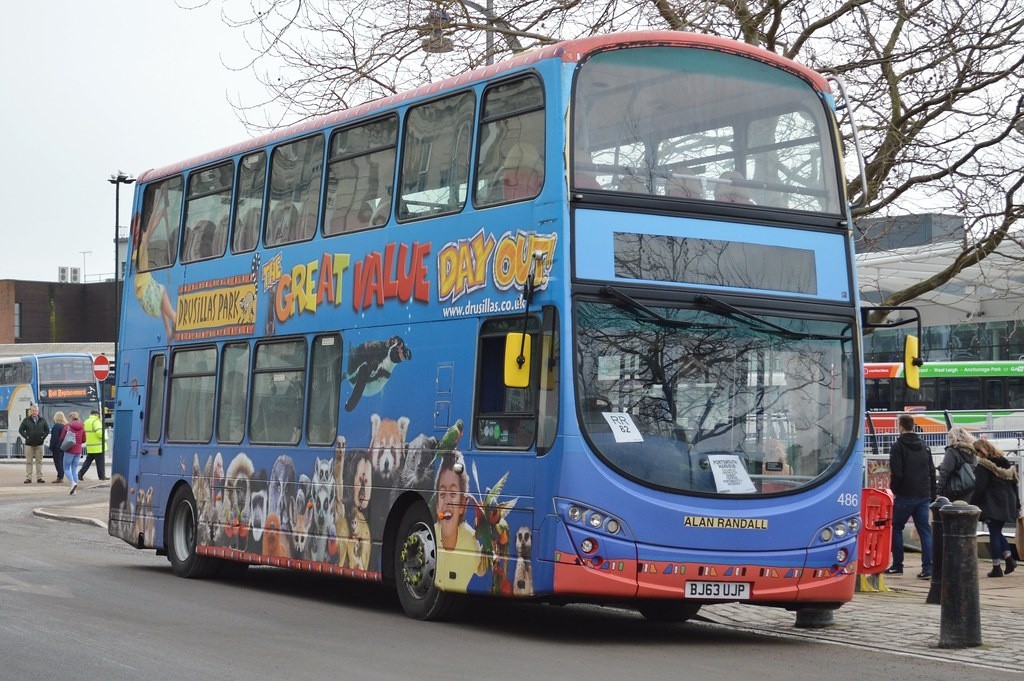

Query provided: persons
[60,412,86,495]
[937,427,979,503]
[49,411,69,483]
[973,440,1021,577]
[502,142,756,208]
[131,183,177,344]
[267,171,298,245]
[78,410,110,481]
[218,365,335,443]
[434,451,501,592]
[19,404,50,483]
[884,415,937,579]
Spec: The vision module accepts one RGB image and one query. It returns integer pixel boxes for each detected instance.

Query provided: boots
[1004,556,1017,574]
[987,564,1003,577]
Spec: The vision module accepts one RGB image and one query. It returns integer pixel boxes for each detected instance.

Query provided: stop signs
[92,355,109,381]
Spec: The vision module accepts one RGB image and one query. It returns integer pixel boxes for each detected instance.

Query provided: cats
[367,413,411,479]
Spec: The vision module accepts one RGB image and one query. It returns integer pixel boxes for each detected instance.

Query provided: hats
[91,410,100,414]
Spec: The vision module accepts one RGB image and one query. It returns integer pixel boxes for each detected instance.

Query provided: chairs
[640,396,688,441]
[141,141,774,270]
[168,390,323,442]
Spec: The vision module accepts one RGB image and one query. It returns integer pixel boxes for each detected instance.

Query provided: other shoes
[37,479,45,483]
[69,482,77,494]
[78,476,83,480]
[24,479,31,483]
[68,491,76,494]
[100,476,110,480]
[52,480,63,483]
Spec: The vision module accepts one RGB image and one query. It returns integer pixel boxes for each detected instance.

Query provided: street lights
[79,251,92,283]
[108,168,137,379]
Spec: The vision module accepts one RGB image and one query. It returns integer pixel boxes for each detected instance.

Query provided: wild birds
[427,418,463,464]
[465,462,521,577]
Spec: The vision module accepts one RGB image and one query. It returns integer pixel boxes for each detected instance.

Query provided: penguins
[340,336,411,412]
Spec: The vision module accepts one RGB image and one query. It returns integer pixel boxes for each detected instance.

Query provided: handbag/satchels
[945,446,977,502]
[61,425,76,451]
[1015,516,1024,560]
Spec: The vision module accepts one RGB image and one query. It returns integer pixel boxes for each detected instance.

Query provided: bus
[860,361,1024,448]
[107,30,923,624]
[0,354,114,457]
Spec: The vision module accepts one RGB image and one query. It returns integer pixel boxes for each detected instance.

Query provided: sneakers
[884,566,903,573]
[917,572,932,580]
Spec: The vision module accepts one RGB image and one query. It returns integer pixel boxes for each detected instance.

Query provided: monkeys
[262,513,289,558]
